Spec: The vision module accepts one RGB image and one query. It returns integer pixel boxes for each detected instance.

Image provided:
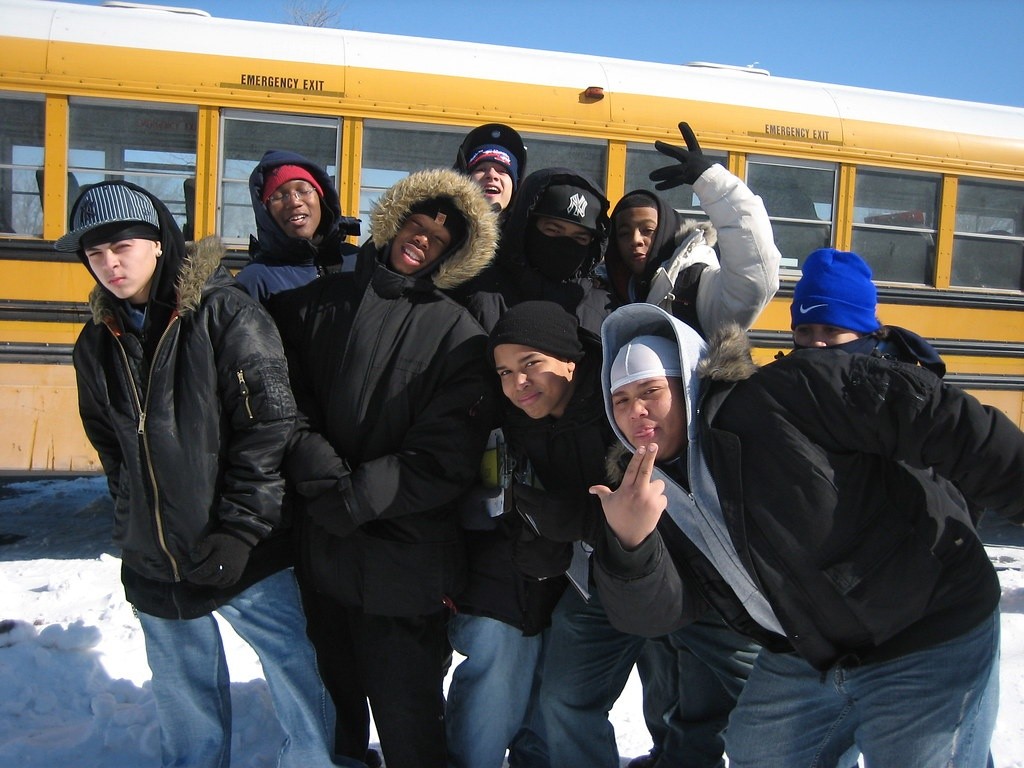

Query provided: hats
[465,143,519,192]
[609,336,682,393]
[408,197,465,242]
[54,185,160,253]
[648,121,716,191]
[262,164,324,204]
[789,247,881,335]
[489,299,587,363]
[533,179,601,236]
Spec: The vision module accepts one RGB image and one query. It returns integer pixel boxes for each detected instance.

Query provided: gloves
[514,483,587,542]
[185,530,253,589]
[296,477,359,538]
[453,486,503,531]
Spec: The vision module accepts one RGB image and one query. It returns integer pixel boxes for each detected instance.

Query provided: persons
[53,181,339,768]
[234,120,1024,768]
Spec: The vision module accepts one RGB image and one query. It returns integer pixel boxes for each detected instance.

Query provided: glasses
[268,187,316,205]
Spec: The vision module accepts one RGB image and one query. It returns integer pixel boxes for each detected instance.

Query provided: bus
[0,0,1024,480]
